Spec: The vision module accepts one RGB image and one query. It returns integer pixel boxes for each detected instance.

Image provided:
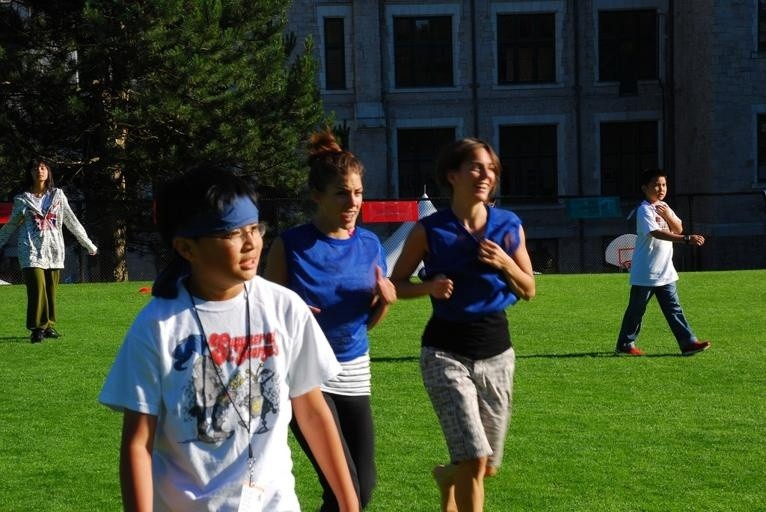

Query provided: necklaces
[185,273,260,489]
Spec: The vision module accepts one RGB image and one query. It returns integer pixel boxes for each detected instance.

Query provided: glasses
[187,224,266,243]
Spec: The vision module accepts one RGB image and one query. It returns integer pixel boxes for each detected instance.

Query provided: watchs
[683,235,691,245]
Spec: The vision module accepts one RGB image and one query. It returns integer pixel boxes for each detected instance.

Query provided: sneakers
[614,345,645,357]
[681,338,711,356]
[43,326,61,339]
[29,327,45,343]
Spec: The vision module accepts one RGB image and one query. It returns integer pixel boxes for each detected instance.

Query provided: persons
[269,130,397,512]
[0,156,99,343]
[389,136,536,512]
[98,167,361,512]
[616,167,712,356]
